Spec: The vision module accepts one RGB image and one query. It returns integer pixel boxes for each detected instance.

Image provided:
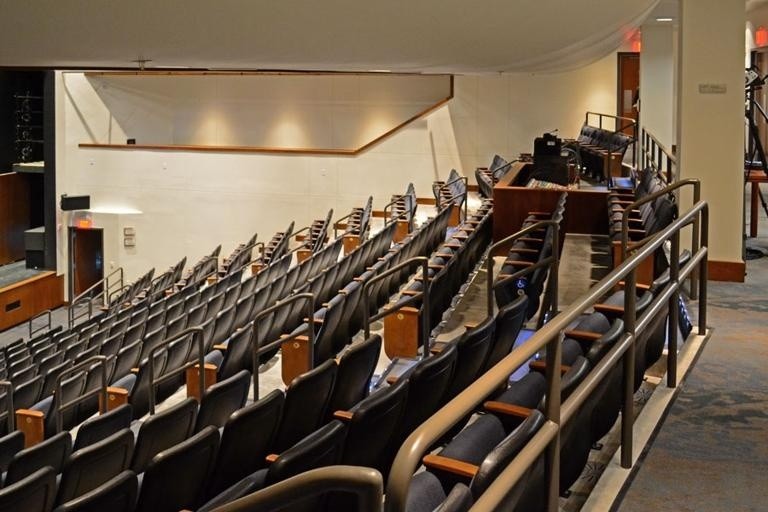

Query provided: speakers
[61,196,90,210]
[15,88,44,163]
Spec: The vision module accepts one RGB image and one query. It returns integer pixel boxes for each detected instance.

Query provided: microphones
[549,128,558,134]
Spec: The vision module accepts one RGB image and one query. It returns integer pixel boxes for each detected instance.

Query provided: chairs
[1,122,680,511]
